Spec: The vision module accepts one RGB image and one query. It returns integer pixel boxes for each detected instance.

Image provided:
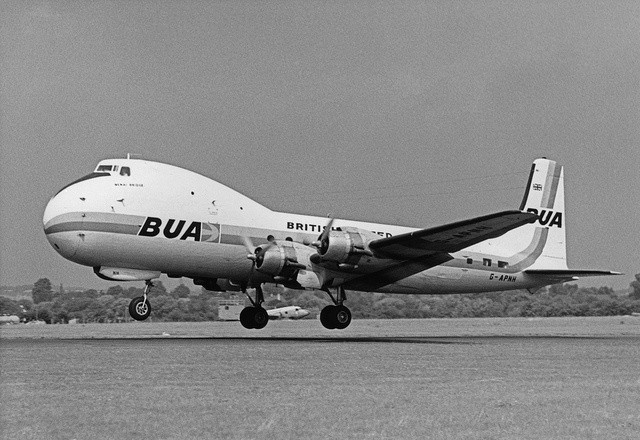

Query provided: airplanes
[265,306,310,320]
[43,153,625,329]
